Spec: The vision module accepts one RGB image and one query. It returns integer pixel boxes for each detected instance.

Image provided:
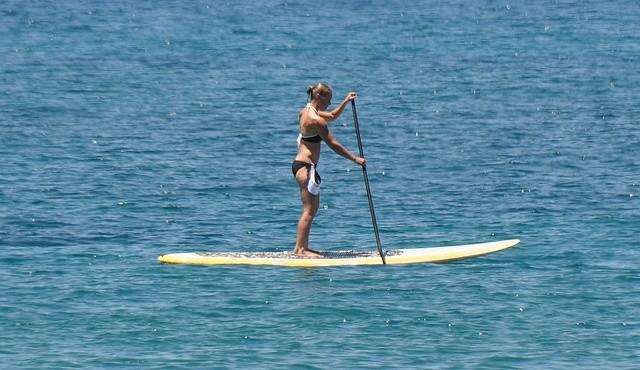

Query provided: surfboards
[157,238,521,267]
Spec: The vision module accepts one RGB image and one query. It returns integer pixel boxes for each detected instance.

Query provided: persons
[292,83,366,258]
